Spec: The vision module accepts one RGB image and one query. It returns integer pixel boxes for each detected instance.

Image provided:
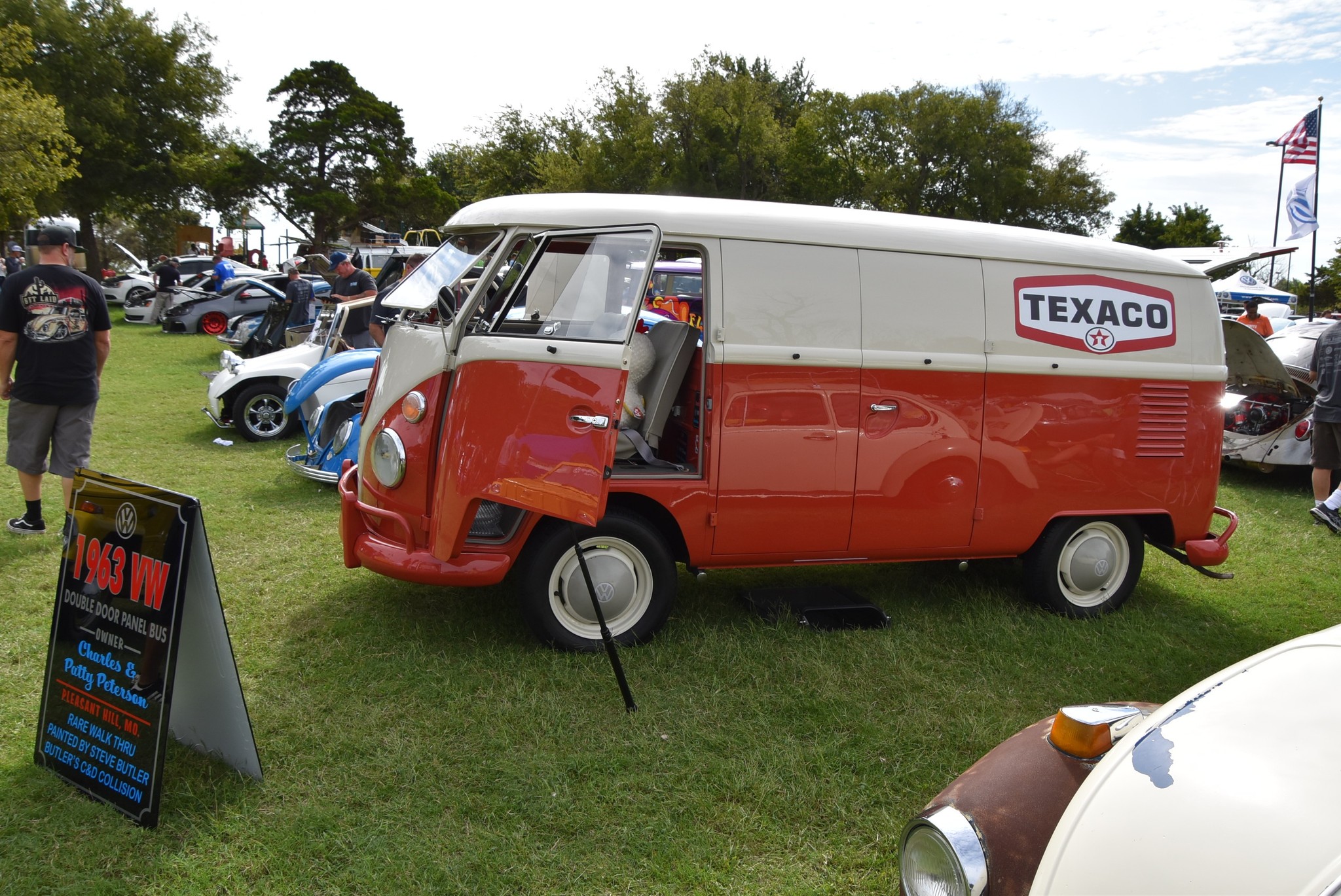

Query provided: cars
[895,624,1341,896]
[0,207,532,361]
[284,301,711,493]
[200,288,524,445]
[1221,302,1341,335]
[1221,321,1341,475]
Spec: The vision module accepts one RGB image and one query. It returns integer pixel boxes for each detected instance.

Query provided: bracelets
[348,296,350,301]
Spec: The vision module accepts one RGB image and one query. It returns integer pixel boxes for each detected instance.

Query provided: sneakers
[6,512,47,535]
[1309,502,1341,536]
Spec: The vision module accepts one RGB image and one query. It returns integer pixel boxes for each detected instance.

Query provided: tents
[1211,269,1297,316]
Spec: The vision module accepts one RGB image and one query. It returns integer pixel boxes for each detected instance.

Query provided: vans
[339,192,1298,711]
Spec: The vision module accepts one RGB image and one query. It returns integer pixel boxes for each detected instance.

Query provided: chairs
[612,319,702,476]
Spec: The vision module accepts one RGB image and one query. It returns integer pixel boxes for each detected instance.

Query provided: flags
[1285,172,1320,241]
[1275,108,1318,165]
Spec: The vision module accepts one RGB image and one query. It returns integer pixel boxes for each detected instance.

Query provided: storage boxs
[360,230,401,243]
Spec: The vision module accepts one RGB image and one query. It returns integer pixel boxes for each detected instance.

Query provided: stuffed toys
[606,317,657,430]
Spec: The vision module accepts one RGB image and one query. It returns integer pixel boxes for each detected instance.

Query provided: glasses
[408,263,415,269]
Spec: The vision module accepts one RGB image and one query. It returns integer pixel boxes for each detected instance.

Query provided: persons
[6,234,18,253]
[212,255,235,294]
[0,224,112,536]
[483,252,493,271]
[148,258,182,325]
[262,255,268,270]
[1309,319,1341,535]
[1235,302,1274,339]
[284,267,316,330]
[186,243,202,256]
[319,251,378,349]
[1243,301,1248,309]
[509,253,518,269]
[5,245,26,279]
[0,258,7,289]
[367,253,438,349]
[1319,309,1332,319]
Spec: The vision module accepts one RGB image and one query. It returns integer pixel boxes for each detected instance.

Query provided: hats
[288,267,299,274]
[37,225,89,254]
[12,245,26,252]
[171,258,182,264]
[327,252,347,272]
